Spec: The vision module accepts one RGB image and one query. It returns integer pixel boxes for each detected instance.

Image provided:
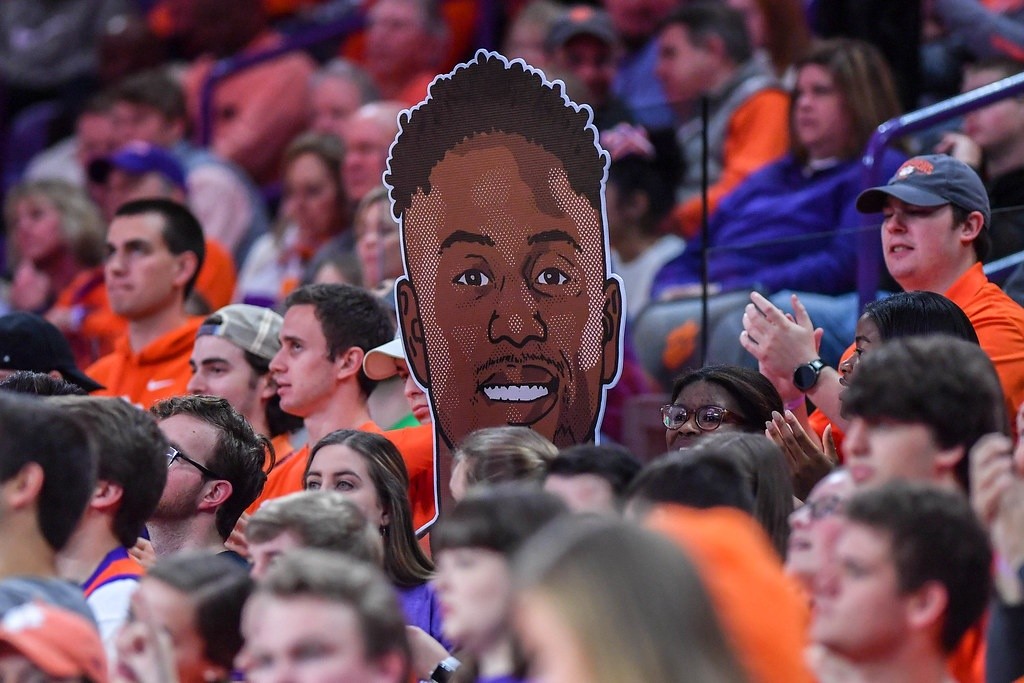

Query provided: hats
[361,326,405,380]
[107,71,189,113]
[0,312,106,393]
[945,14,1024,65]
[195,303,285,362]
[856,153,992,228]
[85,138,186,184]
[542,6,615,47]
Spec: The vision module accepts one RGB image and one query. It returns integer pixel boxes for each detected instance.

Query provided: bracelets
[783,394,805,412]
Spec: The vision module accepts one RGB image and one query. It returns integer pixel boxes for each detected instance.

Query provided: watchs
[430,656,462,683]
[793,359,827,392]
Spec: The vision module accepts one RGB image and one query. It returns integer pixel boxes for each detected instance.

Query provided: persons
[0,0,1024,683]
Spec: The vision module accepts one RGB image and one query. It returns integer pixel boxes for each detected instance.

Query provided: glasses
[795,494,846,519]
[566,48,609,67]
[659,402,747,432]
[164,447,221,480]
